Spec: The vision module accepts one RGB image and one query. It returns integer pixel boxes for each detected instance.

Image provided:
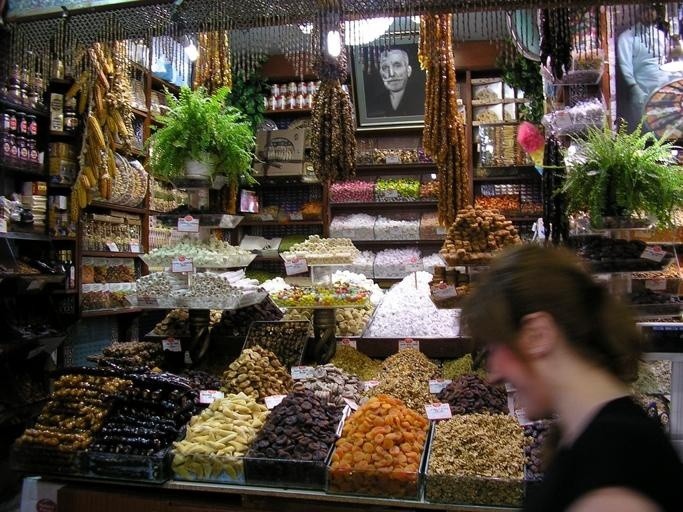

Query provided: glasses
[464,322,507,377]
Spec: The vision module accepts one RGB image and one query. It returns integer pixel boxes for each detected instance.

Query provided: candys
[134,147,523,339]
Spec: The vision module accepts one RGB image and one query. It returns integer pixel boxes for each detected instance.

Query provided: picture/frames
[346,31,426,133]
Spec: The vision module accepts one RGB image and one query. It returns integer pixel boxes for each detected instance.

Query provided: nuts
[171,344,524,499]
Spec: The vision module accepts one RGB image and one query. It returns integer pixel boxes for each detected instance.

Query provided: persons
[615,3,672,130]
[367,49,425,119]
[455,242,682,511]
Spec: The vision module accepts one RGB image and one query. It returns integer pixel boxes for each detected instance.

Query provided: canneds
[0,64,80,163]
[263,80,322,111]
